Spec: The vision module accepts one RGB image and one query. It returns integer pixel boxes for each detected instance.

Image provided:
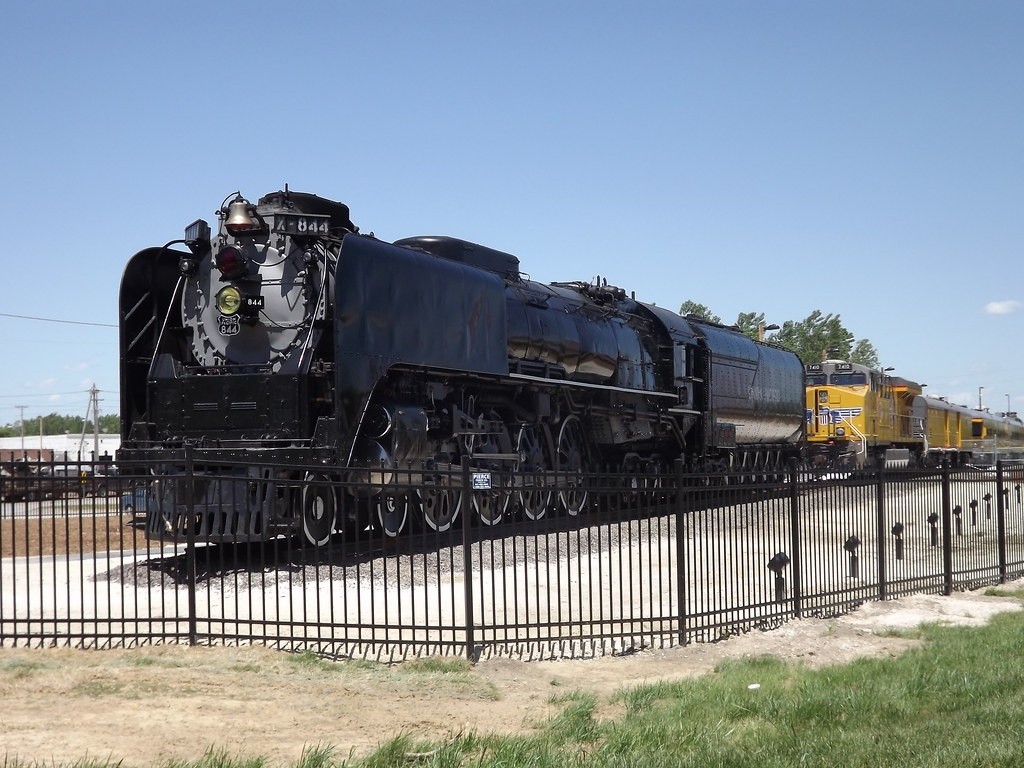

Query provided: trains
[108,181,1023,549]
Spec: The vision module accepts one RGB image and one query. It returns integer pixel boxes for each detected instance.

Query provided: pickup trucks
[78,467,122,498]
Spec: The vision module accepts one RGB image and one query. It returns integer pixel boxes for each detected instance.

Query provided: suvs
[47,466,96,495]
